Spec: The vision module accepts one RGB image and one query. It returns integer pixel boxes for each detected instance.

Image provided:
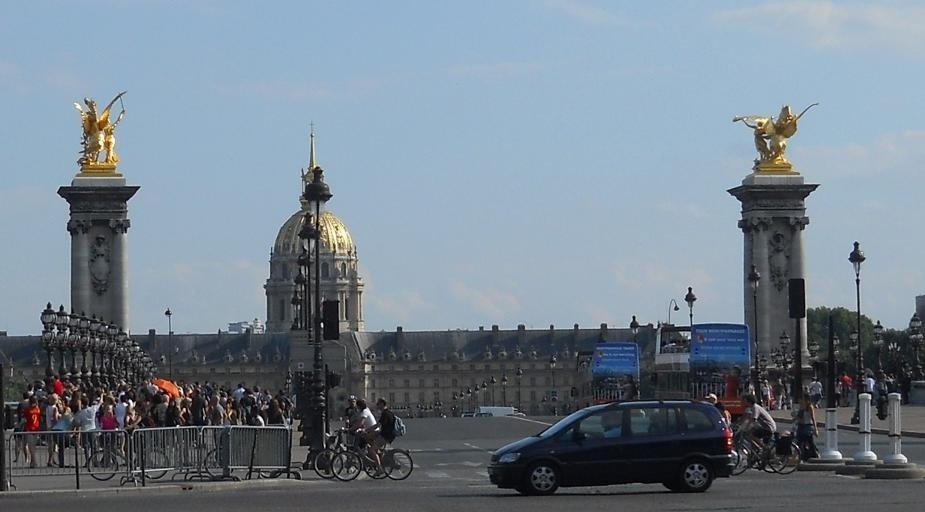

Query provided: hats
[704,393,717,404]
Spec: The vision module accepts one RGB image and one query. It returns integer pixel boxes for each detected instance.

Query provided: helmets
[348,394,358,400]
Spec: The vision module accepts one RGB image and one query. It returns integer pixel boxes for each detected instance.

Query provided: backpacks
[392,416,407,438]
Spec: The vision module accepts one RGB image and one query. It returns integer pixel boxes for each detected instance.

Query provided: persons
[584,412,621,439]
[7,379,295,468]
[345,395,398,478]
[615,373,638,403]
[705,365,913,472]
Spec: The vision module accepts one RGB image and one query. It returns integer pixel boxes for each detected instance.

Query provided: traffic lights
[787,277,805,319]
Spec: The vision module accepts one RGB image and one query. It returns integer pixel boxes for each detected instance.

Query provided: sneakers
[373,469,385,477]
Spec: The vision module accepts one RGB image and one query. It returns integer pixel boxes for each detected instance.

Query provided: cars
[459,406,528,418]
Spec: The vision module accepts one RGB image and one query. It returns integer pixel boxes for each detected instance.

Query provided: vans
[488,401,737,496]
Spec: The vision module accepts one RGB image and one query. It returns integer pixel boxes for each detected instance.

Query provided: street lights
[548,352,561,415]
[283,165,334,468]
[685,286,698,402]
[516,367,522,413]
[390,379,488,418]
[165,307,174,380]
[630,315,643,400]
[848,241,867,422]
[490,376,497,407]
[501,373,507,407]
[7,300,159,404]
[668,299,679,343]
[598,332,606,343]
[748,264,765,408]
[753,310,925,409]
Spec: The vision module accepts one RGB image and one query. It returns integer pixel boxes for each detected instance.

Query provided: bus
[571,341,640,413]
[654,322,753,420]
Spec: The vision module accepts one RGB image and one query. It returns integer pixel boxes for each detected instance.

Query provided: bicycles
[313,417,414,483]
[725,420,803,476]
[87,432,171,481]
[205,447,234,482]
